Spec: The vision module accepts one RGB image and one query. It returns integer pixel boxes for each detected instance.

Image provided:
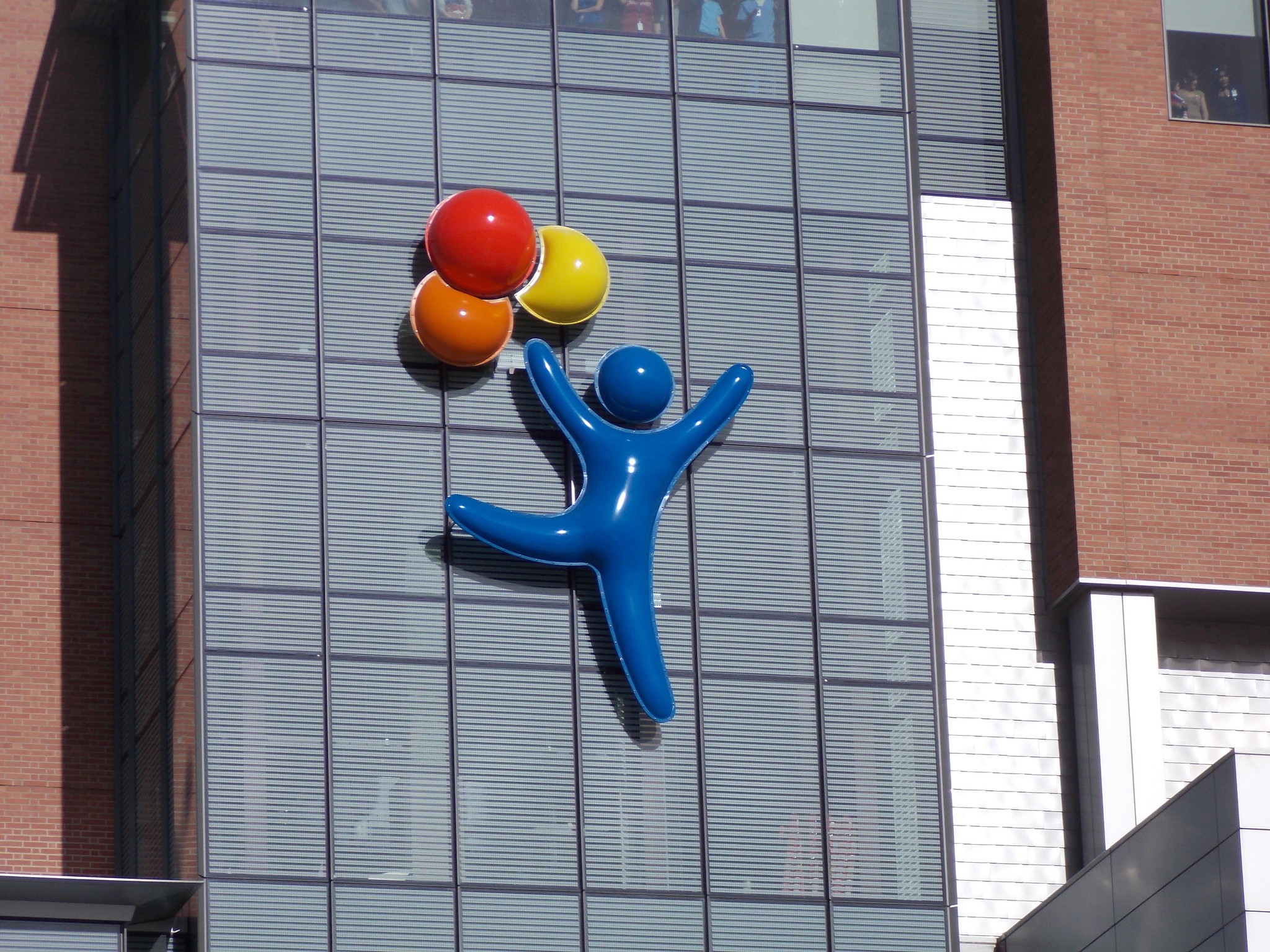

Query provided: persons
[1170,62,1245,124]
[372,0,783,42]
